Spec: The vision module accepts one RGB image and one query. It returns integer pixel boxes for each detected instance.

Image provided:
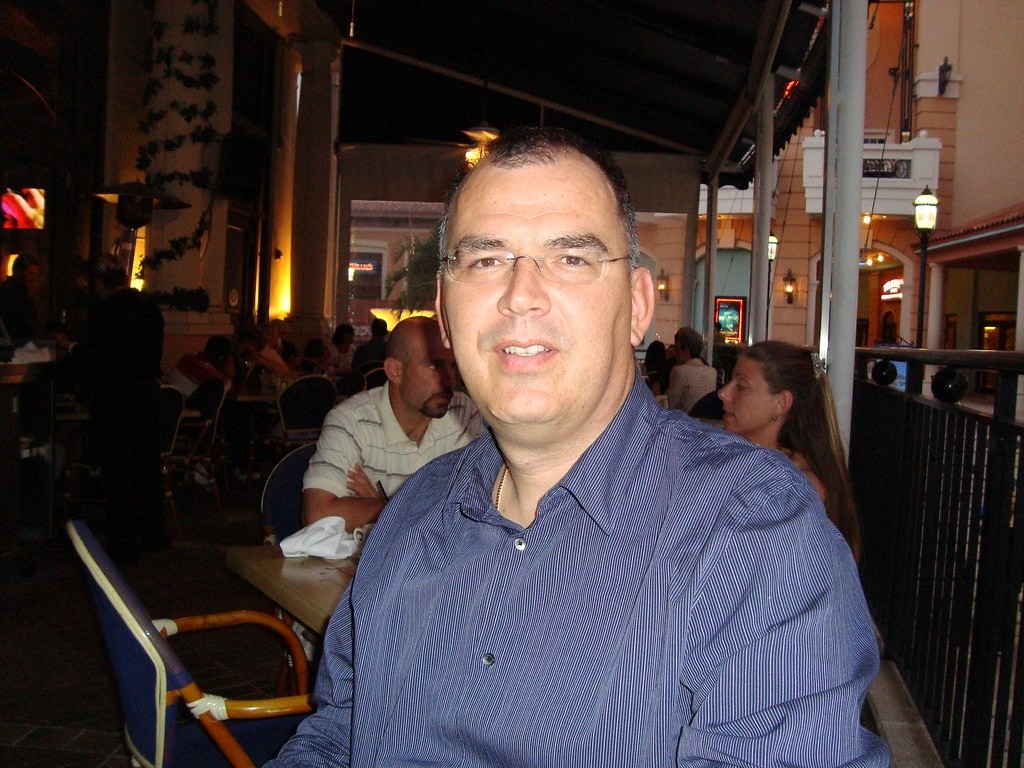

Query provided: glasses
[438,243,631,285]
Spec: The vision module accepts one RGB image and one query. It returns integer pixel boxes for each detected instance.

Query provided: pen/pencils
[375,481,389,506]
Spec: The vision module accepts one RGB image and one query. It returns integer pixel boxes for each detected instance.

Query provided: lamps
[92,179,152,204]
[460,84,499,171]
[656,268,670,302]
[782,267,796,304]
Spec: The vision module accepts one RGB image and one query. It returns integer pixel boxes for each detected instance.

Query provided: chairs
[51,358,729,768]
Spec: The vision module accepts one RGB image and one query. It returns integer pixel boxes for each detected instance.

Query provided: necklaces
[496,466,509,511]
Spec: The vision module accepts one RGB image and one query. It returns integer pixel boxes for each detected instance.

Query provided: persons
[718,340,861,564]
[1,254,164,516]
[260,127,893,768]
[202,319,390,497]
[302,317,487,533]
[644,326,726,431]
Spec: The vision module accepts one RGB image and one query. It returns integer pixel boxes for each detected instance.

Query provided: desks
[54,407,202,459]
[217,542,360,647]
